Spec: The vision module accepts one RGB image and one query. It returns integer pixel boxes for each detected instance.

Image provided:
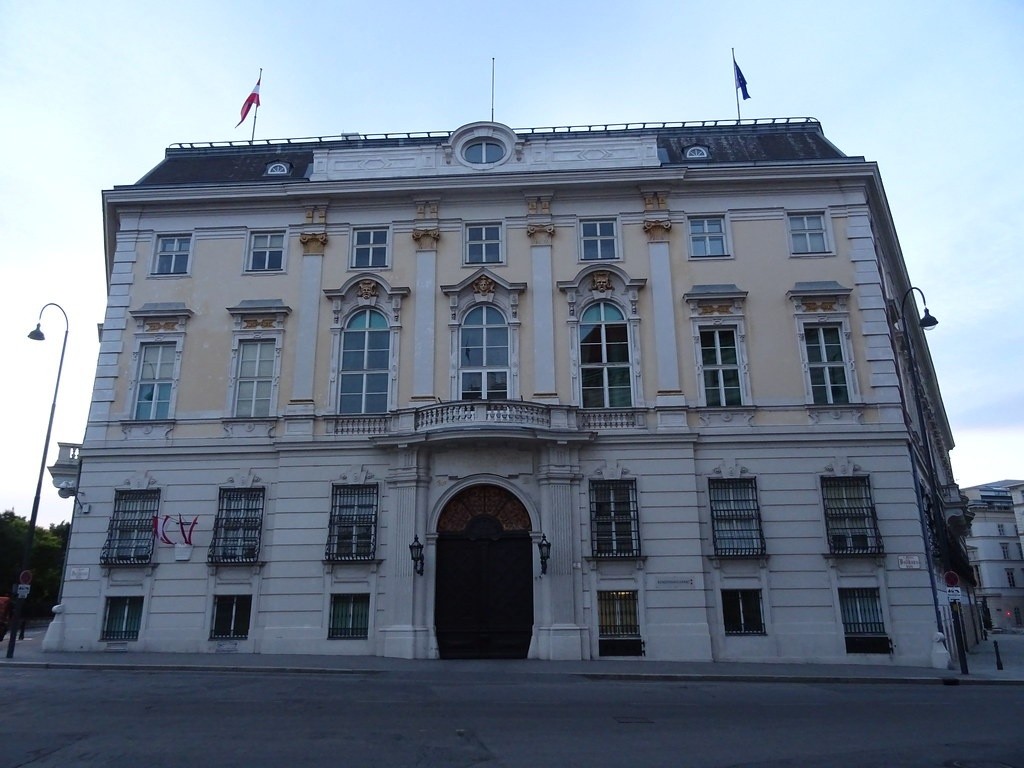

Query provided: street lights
[6,302,69,658]
[901,286,970,675]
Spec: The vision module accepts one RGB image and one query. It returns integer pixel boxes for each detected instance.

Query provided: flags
[234,68,263,129]
[734,54,751,100]
